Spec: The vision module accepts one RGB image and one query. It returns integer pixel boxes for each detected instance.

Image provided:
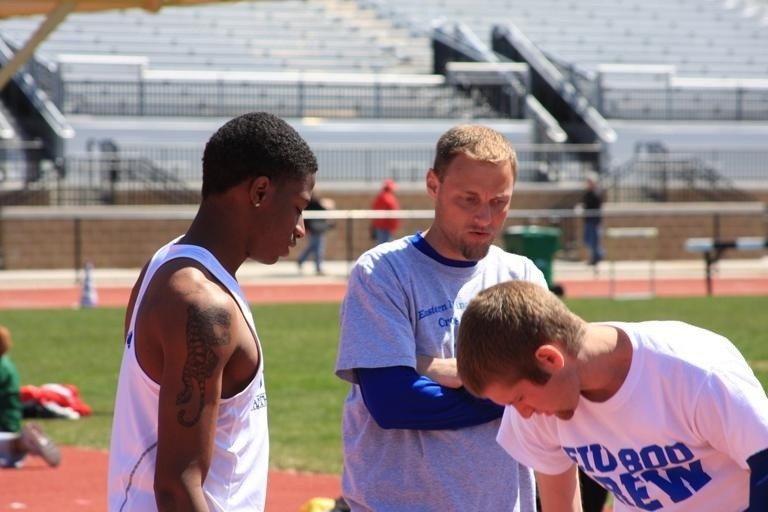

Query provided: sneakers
[19,421,59,466]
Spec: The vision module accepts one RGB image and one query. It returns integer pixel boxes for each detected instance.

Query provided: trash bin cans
[501,225,564,298]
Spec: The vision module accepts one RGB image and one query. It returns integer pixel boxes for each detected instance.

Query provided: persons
[329,124,551,512]
[0,421,62,468]
[572,167,604,266]
[106,110,319,512]
[296,194,333,274]
[455,278,767,512]
[369,179,400,242]
[0,325,23,469]
